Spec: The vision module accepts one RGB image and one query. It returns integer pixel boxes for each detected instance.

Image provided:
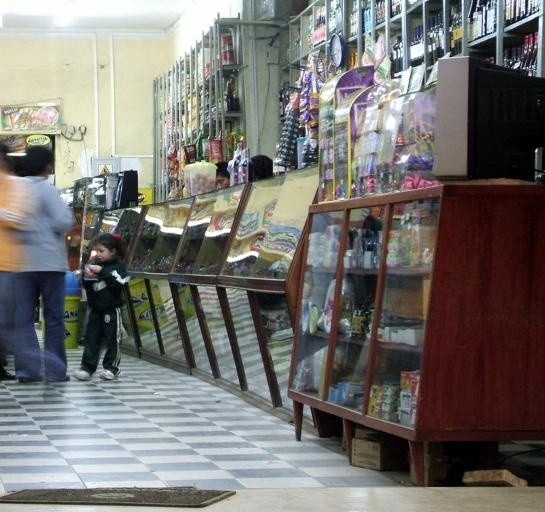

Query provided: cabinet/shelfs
[286,184,544,487]
[153,13,246,204]
[280,0,545,169]
[1,102,60,186]
[83,166,330,438]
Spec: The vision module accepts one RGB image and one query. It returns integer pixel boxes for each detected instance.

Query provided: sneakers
[73,369,92,381]
[99,367,122,380]
[0,366,17,381]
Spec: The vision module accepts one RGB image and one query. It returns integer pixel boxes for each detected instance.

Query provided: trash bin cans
[42,272,83,350]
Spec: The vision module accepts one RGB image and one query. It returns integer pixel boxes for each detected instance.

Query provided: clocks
[329,34,343,67]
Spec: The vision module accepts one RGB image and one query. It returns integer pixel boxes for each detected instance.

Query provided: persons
[74,235,132,380]
[215,162,230,188]
[0,139,17,381]
[1,145,74,384]
[247,154,272,180]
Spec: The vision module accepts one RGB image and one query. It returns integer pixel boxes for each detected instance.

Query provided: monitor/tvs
[433,56,544,178]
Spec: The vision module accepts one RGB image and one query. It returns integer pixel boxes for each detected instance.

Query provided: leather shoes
[20,371,43,384]
[46,374,72,385]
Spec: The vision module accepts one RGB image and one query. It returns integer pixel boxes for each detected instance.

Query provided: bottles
[313,5,326,45]
[392,37,405,72]
[468,2,497,42]
[426,11,448,65]
[225,80,234,113]
[277,81,290,116]
[390,0,399,16]
[328,1,344,37]
[349,1,358,36]
[520,32,539,75]
[503,0,537,23]
[376,3,384,24]
[450,5,464,55]
[409,25,428,68]
[364,2,373,32]
